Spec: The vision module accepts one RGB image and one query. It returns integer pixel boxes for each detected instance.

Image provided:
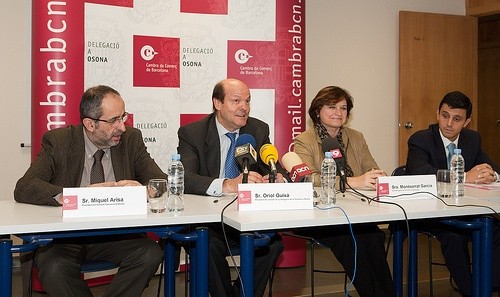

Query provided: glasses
[85,112,129,126]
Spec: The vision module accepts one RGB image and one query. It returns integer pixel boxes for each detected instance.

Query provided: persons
[294,86,397,297]
[14,85,168,297]
[405,91,500,297]
[177,78,294,297]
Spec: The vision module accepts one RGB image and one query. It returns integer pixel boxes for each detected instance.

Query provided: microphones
[260,143,279,171]
[323,138,346,178]
[282,152,312,183]
[235,134,258,167]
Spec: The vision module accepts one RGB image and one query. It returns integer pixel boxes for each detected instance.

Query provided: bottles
[320,152,336,206]
[450,149,465,197]
[167,154,185,212]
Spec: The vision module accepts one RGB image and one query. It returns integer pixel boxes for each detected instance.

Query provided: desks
[0,196,221,297]
[184,184,500,297]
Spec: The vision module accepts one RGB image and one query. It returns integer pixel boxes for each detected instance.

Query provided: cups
[148,179,168,213]
[305,175,321,206]
[437,170,453,198]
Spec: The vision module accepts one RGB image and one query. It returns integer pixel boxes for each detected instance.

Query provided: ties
[225,132,239,179]
[90,150,105,184]
[446,143,456,170]
